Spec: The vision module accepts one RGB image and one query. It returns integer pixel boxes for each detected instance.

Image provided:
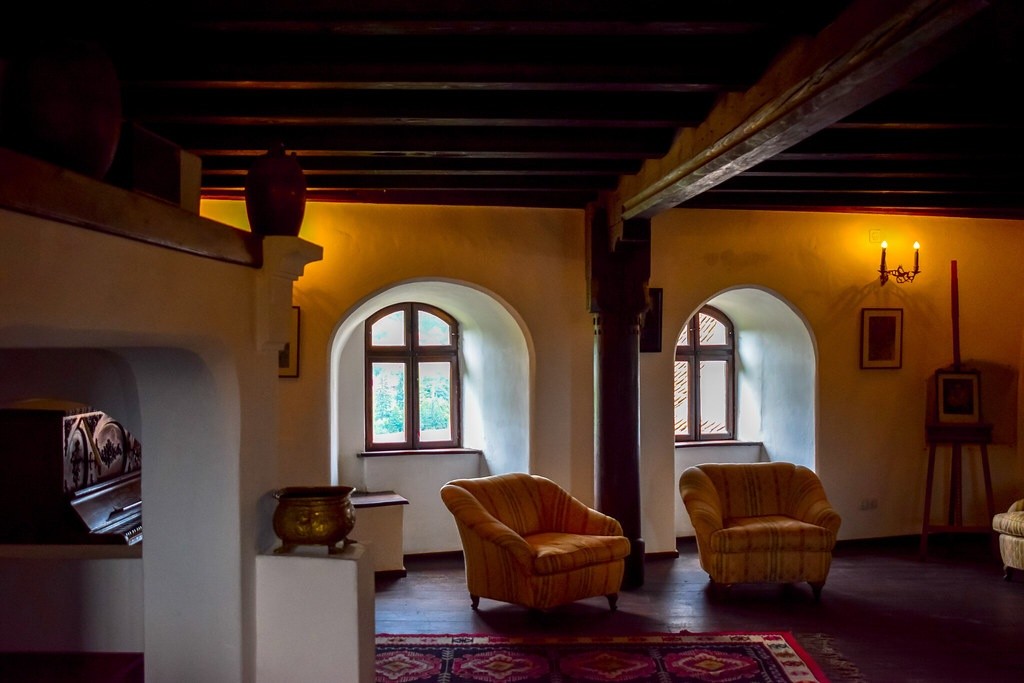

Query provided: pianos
[0,408,143,544]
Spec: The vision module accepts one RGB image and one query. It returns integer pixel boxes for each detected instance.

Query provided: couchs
[441,474,631,634]
[678,462,844,604]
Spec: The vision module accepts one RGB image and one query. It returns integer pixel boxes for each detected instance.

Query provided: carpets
[375,631,868,683]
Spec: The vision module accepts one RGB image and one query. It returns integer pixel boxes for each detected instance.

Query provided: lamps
[877,240,922,288]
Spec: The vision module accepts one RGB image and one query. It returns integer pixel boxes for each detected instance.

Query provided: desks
[915,422,1002,563]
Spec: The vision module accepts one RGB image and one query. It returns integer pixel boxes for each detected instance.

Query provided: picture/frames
[279,305,300,379]
[935,368,984,428]
[859,307,903,370]
